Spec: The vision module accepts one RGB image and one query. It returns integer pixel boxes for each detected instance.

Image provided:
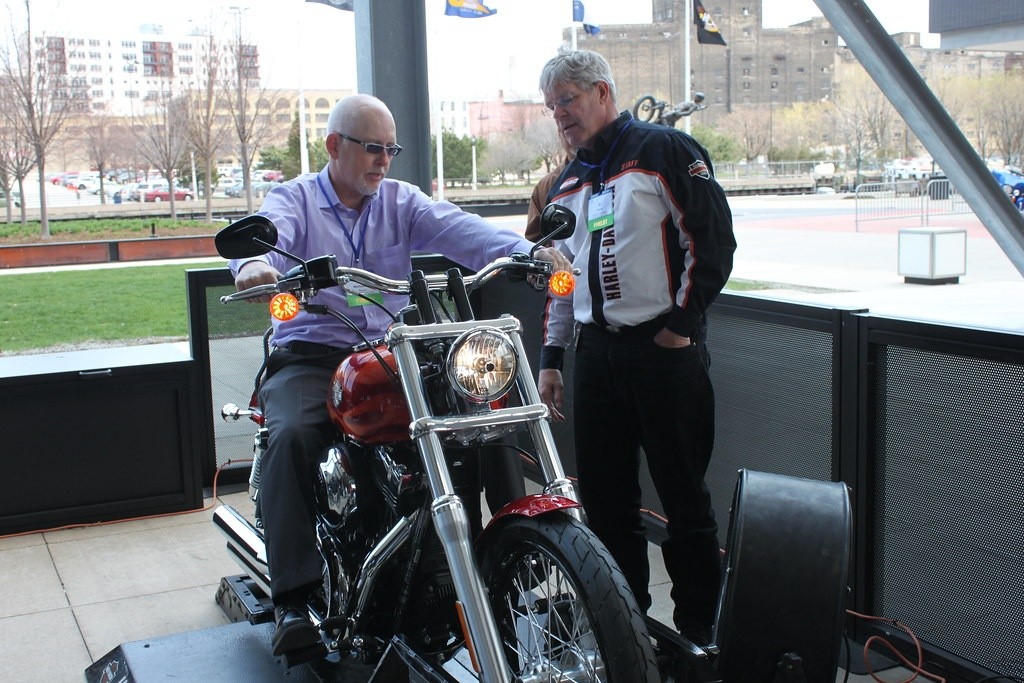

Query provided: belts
[278,340,337,356]
[595,324,661,335]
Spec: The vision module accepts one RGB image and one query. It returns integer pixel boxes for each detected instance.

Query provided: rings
[561,256,566,259]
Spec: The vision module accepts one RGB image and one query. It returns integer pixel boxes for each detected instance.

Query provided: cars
[146,185,195,201]
[47,168,177,201]
[192,165,287,199]
[0,192,22,207]
[993,169,1024,209]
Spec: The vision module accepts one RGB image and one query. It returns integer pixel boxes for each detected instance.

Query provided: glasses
[335,132,403,156]
[540,89,580,120]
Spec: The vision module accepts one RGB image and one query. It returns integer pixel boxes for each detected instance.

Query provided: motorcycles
[212,203,665,683]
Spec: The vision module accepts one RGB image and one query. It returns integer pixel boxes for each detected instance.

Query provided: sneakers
[271,603,322,657]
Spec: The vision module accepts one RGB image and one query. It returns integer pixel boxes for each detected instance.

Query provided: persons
[225,93,577,659]
[525,51,724,652]
